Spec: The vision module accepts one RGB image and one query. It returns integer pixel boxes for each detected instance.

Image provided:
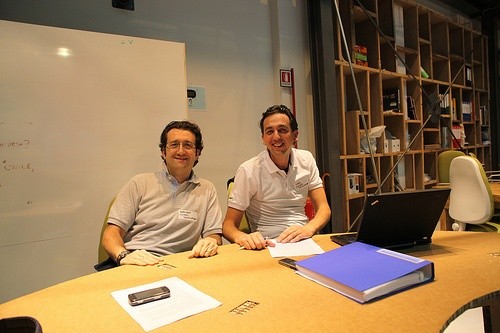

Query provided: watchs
[116,250,131,266]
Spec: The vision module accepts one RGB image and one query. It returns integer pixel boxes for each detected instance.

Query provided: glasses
[163,141,198,150]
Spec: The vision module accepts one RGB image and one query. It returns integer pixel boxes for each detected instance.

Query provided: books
[463,65,472,88]
[382,88,400,113]
[440,90,487,148]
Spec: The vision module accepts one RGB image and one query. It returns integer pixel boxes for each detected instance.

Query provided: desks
[0,232,500,333]
[432,181,500,231]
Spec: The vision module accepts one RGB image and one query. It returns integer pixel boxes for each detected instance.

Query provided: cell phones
[279,257,297,270]
[128,287,170,305]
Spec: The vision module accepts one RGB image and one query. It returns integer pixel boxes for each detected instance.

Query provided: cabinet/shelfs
[316,0,492,234]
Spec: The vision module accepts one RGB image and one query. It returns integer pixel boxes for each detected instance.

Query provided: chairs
[437,151,467,185]
[449,156,500,233]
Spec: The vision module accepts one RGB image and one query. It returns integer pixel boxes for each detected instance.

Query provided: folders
[294,242,434,304]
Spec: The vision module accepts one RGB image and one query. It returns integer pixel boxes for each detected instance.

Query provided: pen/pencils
[239,236,266,250]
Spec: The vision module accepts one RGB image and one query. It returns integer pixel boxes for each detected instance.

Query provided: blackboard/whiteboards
[0,20,189,304]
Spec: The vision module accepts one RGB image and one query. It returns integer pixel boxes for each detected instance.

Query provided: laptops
[330,187,452,251]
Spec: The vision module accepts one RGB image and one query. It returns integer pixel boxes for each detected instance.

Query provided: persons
[221,104,331,251]
[100,120,223,267]
